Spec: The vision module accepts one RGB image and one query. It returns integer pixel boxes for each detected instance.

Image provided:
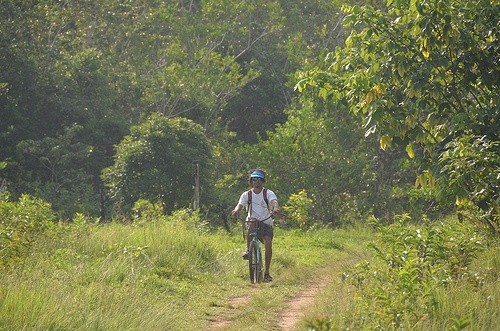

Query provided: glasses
[252,178,264,182]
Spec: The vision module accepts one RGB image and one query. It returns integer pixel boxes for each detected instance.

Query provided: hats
[250,172,265,180]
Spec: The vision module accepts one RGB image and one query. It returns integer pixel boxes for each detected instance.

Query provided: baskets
[243,220,265,237]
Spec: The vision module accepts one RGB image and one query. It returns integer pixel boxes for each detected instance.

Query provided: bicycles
[232,210,286,285]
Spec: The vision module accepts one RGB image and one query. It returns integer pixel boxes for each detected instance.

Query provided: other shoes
[263,274,272,281]
[243,248,250,260]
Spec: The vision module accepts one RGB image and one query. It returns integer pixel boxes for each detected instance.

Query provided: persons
[232,169,281,282]
